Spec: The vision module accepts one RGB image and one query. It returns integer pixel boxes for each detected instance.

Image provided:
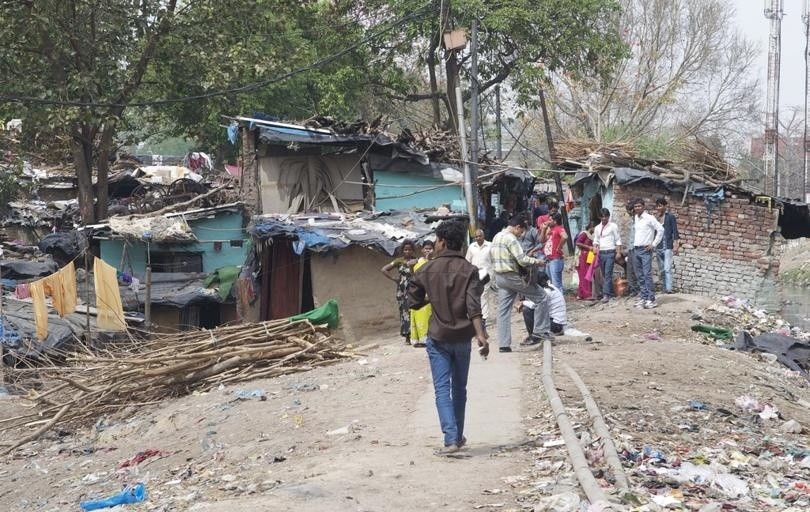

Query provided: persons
[407,219,489,454]
[382,229,491,348]
[573,198,678,309]
[467,198,568,353]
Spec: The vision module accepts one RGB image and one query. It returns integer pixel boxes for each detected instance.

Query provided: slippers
[433,434,466,456]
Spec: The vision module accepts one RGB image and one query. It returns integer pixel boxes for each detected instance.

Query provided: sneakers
[521,332,555,345]
[500,346,512,352]
[601,294,609,302]
[634,298,658,309]
